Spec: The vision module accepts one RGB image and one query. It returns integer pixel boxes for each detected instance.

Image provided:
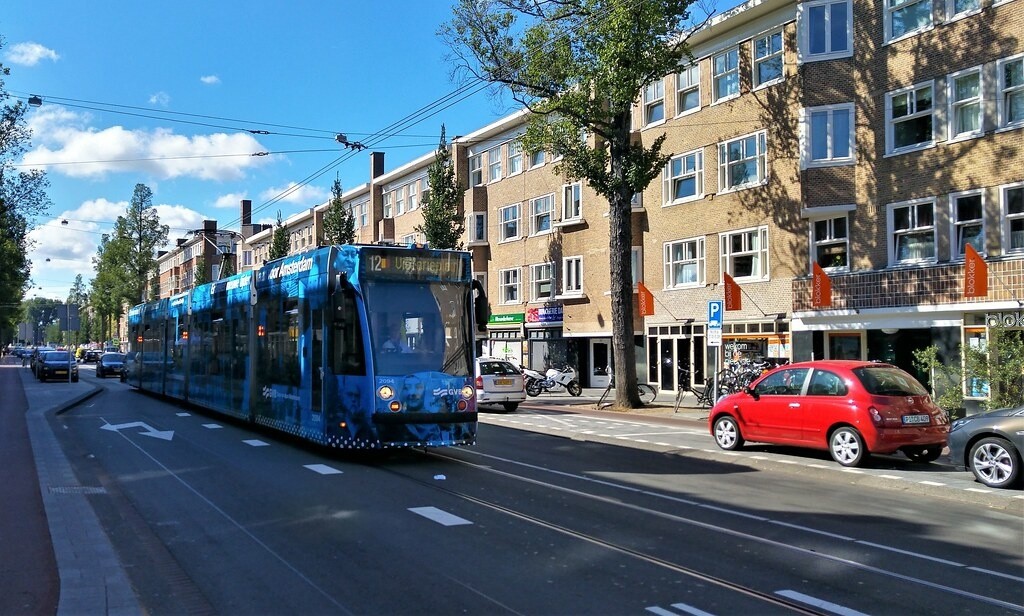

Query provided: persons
[381,324,415,354]
[0,345,8,364]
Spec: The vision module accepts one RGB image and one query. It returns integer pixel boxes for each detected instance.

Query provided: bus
[126,229,489,450]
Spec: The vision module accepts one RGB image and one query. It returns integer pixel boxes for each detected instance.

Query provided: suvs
[473,356,527,412]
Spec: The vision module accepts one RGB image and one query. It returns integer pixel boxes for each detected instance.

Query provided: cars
[947,404,1024,489]
[14,347,56,376]
[75,347,121,364]
[119,355,126,383]
[38,351,79,383]
[708,360,950,469]
[96,352,126,379]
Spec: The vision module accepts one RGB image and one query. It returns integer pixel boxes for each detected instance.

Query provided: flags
[637,282,655,317]
[965,245,989,297]
[812,262,832,306]
[723,272,743,311]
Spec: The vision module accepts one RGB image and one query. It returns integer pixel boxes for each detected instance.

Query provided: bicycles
[596,365,657,406]
[673,360,771,413]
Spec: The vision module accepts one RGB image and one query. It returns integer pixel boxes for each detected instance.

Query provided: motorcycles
[518,362,582,397]
